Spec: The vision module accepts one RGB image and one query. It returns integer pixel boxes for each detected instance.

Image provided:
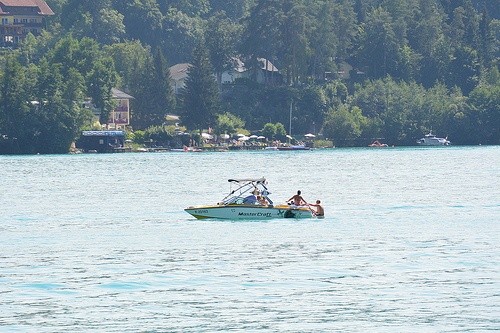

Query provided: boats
[368,140,388,147]
[277,145,310,150]
[415,129,450,146]
[184,177,314,221]
[170,145,202,152]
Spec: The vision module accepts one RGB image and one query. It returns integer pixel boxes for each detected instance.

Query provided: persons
[285,190,324,217]
[257,196,269,206]
